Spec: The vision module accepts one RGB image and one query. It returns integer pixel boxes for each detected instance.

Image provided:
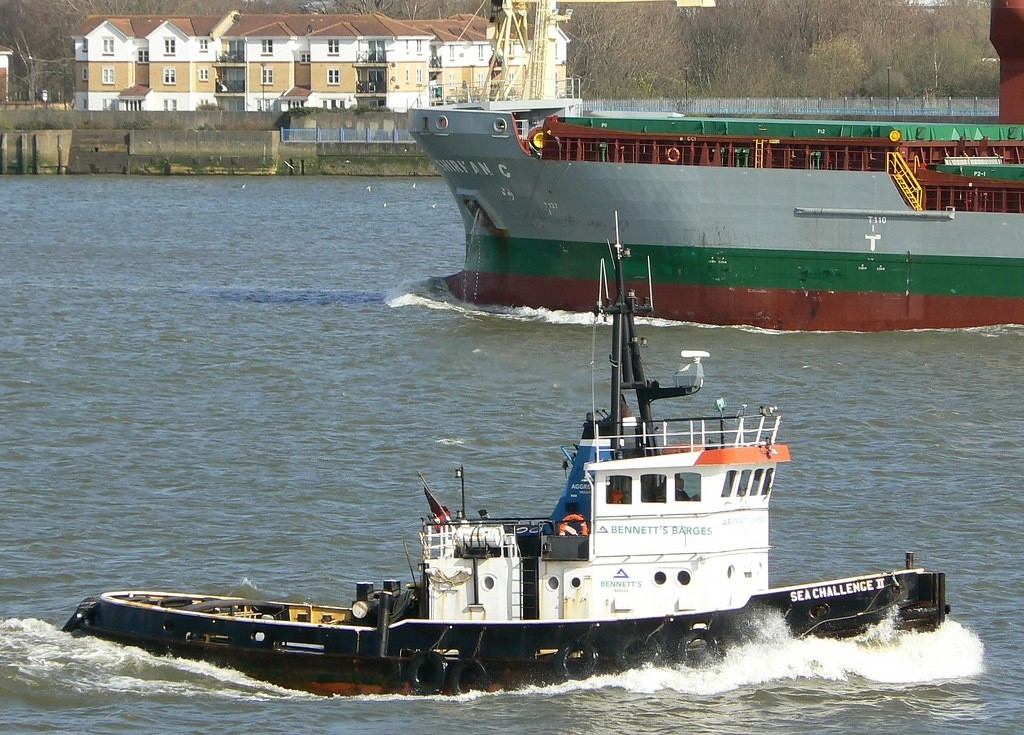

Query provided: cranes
[484,0,575,103]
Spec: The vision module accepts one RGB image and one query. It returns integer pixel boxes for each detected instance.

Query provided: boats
[404,100,1023,337]
[54,209,953,700]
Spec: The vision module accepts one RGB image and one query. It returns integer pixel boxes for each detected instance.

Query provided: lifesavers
[558,514,589,536]
[667,148,680,162]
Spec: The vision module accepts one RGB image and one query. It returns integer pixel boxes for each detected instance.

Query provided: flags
[422,484,451,533]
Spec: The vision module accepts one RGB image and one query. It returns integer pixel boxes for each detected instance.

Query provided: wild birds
[431,203,436,209]
[412,182,416,189]
[366,184,371,192]
[284,161,294,170]
[384,203,387,208]
